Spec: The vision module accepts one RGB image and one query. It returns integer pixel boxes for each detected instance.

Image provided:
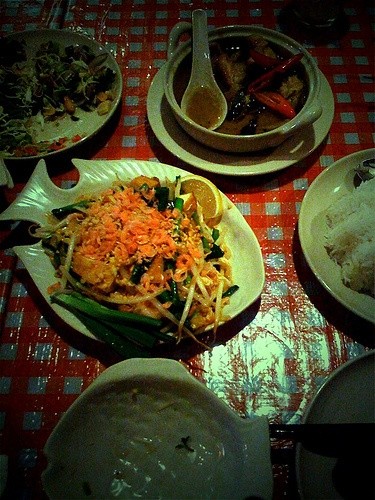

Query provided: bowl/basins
[1,29,122,161]
[162,21,323,153]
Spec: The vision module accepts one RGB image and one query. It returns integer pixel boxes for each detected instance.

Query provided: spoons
[180,9,228,131]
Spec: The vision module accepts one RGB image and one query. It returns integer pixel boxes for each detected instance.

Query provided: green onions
[52,290,175,359]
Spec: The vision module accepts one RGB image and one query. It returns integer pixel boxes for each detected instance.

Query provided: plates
[295,350,375,499]
[42,356,274,499]
[147,63,336,177]
[298,148,374,324]
[1,157,265,346]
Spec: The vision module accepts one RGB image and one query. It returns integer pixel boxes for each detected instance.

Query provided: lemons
[179,174,223,218]
